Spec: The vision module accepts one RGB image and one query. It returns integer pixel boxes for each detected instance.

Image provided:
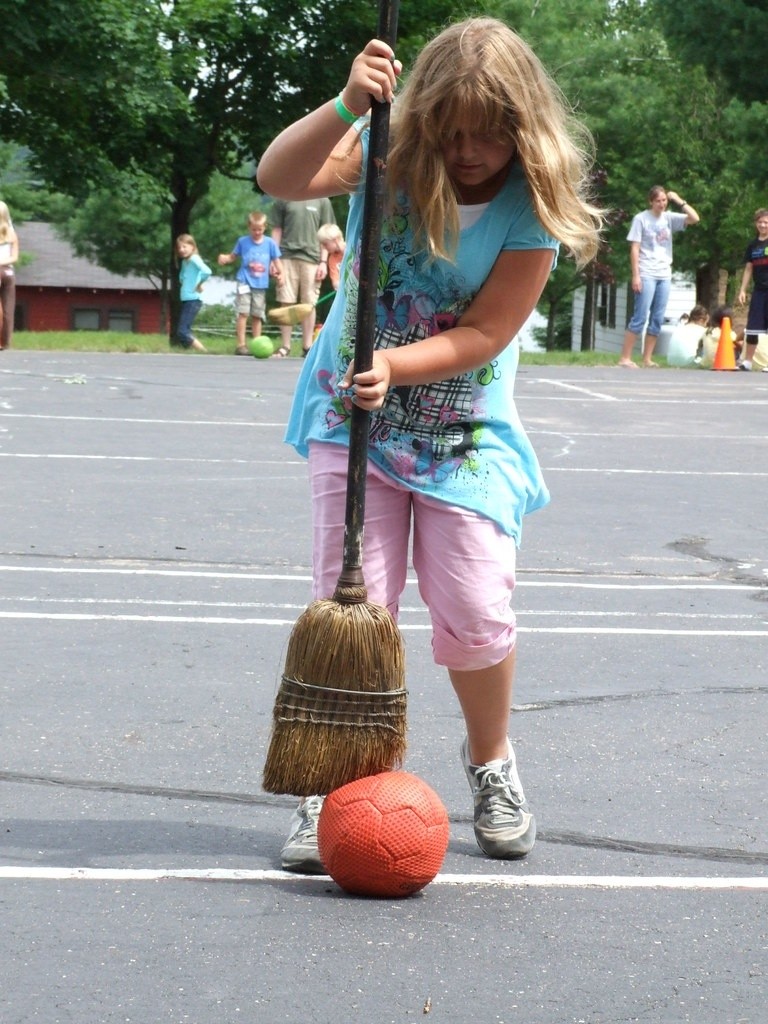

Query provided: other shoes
[235,346,250,355]
[199,348,208,354]
[739,361,752,370]
[620,360,639,368]
[642,362,659,367]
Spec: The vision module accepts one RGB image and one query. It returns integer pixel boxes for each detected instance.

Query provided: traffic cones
[710,317,739,372]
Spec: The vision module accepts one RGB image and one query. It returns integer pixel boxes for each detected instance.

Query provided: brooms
[265,0,404,798]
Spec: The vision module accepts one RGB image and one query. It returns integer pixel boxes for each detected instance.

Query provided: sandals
[272,346,291,358]
[301,348,310,357]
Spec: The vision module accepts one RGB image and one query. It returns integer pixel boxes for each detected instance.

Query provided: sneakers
[281,794,328,871]
[460,733,537,858]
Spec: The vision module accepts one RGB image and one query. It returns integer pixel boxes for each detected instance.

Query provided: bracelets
[680,200,687,208]
[334,92,359,123]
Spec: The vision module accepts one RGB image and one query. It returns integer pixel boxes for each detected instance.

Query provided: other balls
[316,771,452,900]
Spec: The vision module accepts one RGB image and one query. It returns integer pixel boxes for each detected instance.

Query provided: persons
[217,197,347,356]
[617,185,701,368]
[702,305,742,368]
[258,14,607,867]
[667,305,710,366]
[176,234,213,351]
[0,201,19,351]
[737,208,768,372]
